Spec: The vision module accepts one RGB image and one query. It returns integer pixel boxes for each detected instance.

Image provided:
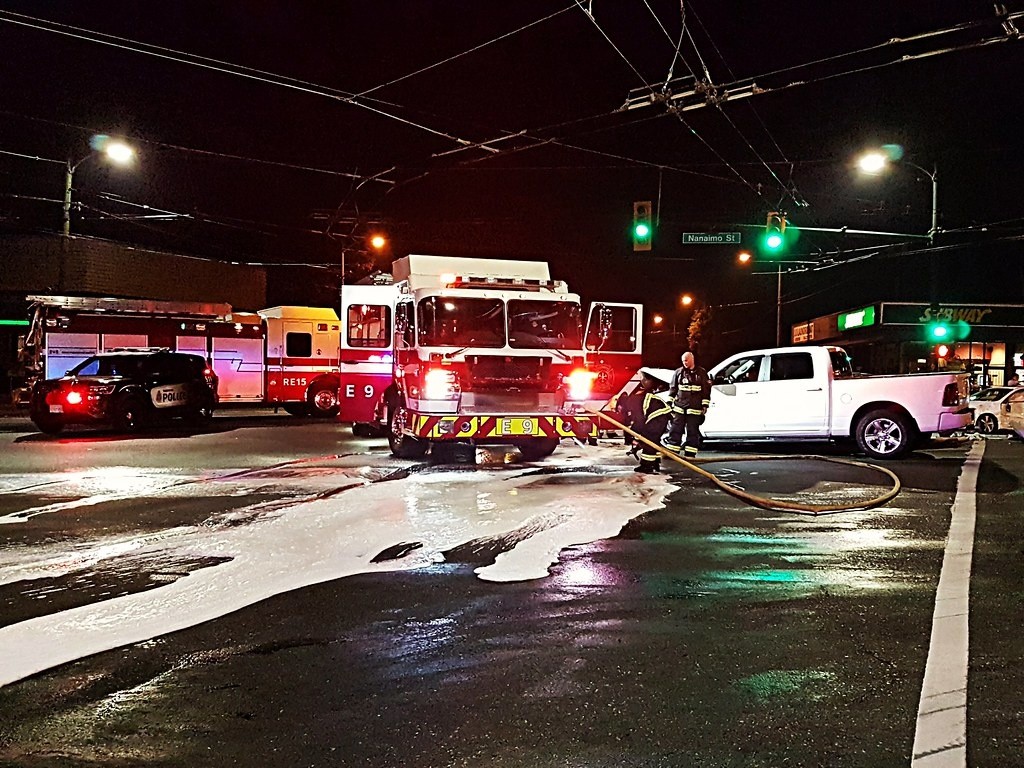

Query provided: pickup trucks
[655,345,975,459]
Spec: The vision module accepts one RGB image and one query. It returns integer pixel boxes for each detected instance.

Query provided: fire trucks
[13,294,340,419]
[337,254,644,466]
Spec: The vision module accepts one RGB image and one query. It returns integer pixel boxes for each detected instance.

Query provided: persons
[660,351,712,461]
[1008,374,1021,387]
[749,367,759,381]
[965,359,974,386]
[610,392,674,475]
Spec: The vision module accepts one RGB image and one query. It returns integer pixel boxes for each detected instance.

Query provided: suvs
[29,345,219,435]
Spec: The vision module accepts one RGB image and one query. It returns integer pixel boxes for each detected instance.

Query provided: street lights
[63,141,134,236]
[861,154,938,247]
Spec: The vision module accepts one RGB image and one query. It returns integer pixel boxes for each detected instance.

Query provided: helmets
[610,391,628,413]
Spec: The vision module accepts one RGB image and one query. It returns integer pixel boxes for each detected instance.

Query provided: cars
[969,386,1024,435]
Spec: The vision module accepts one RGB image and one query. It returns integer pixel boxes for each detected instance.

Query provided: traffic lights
[931,324,951,358]
[766,211,785,253]
[633,201,652,251]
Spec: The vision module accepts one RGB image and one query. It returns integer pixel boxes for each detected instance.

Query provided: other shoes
[634,465,652,473]
[652,463,660,471]
